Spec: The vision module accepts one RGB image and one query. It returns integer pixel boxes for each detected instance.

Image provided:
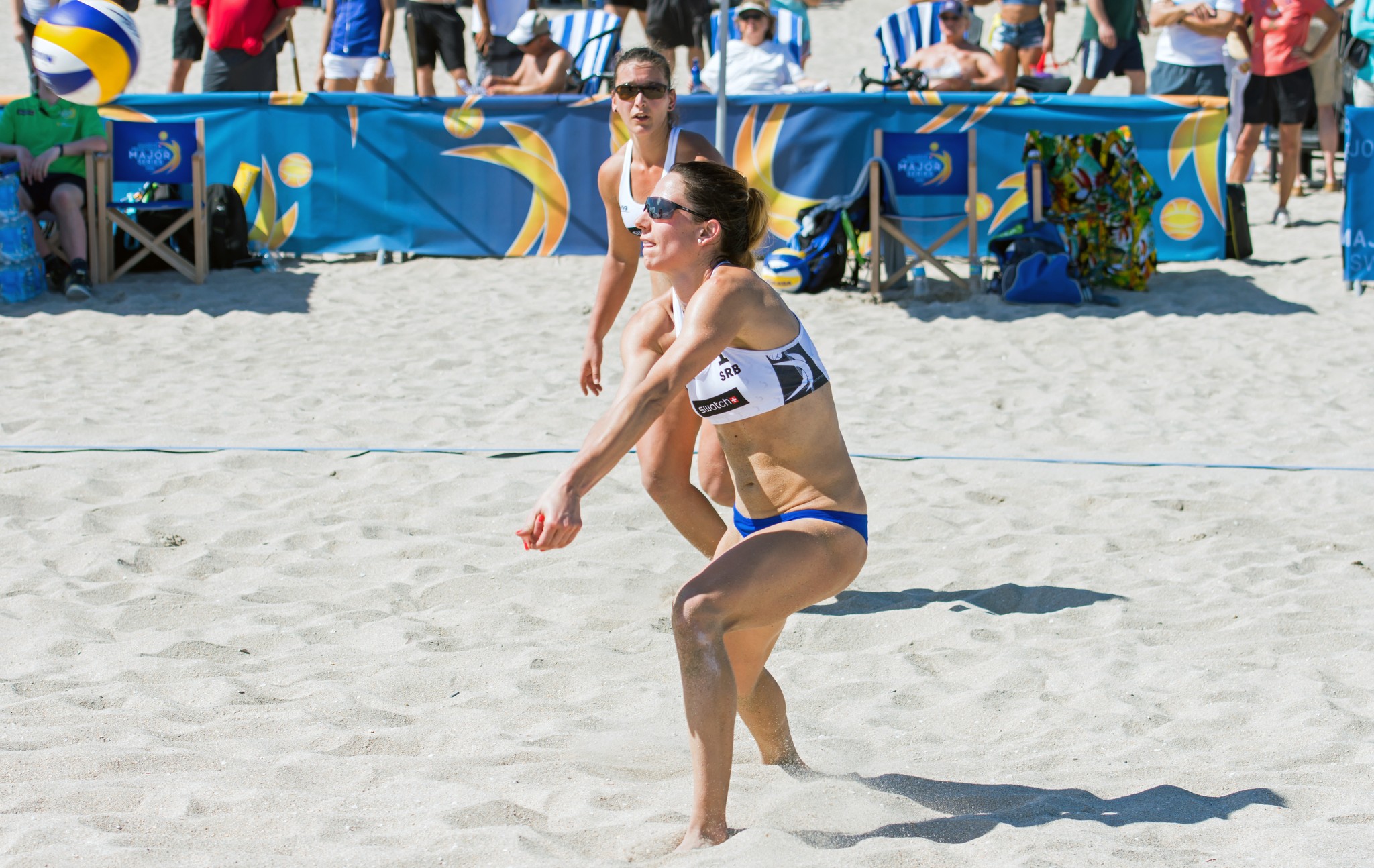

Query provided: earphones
[343,46,349,53]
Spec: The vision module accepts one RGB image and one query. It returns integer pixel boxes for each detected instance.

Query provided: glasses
[738,14,764,21]
[940,13,961,21]
[611,82,671,100]
[643,196,734,232]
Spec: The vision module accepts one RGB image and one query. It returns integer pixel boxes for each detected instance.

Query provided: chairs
[869,129,977,302]
[1021,125,1163,292]
[548,8,622,94]
[710,4,803,66]
[84,117,209,287]
[875,1,982,91]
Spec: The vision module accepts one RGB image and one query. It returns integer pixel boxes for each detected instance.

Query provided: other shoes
[1292,187,1305,197]
[42,252,93,296]
[1273,208,1293,228]
[1322,178,1340,192]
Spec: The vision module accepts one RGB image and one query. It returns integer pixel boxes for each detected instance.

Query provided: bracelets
[56,143,65,157]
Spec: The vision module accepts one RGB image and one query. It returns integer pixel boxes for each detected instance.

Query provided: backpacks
[789,204,848,293]
[185,183,249,269]
[114,182,185,269]
[986,217,1087,305]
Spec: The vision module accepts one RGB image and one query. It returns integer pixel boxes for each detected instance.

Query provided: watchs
[378,52,389,60]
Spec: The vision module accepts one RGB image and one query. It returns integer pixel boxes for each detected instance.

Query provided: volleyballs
[30,0,140,107]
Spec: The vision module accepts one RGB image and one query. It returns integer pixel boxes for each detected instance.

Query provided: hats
[734,0,770,17]
[936,0,963,17]
[506,9,549,46]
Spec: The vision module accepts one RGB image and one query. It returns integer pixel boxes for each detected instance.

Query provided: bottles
[913,261,925,296]
[0,172,44,298]
[247,239,280,272]
[969,253,982,292]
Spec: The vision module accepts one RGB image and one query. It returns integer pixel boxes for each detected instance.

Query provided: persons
[600,0,821,98]
[0,74,110,299]
[13,0,302,99]
[889,0,1374,227]
[514,160,870,852]
[319,0,586,96]
[577,40,737,558]
